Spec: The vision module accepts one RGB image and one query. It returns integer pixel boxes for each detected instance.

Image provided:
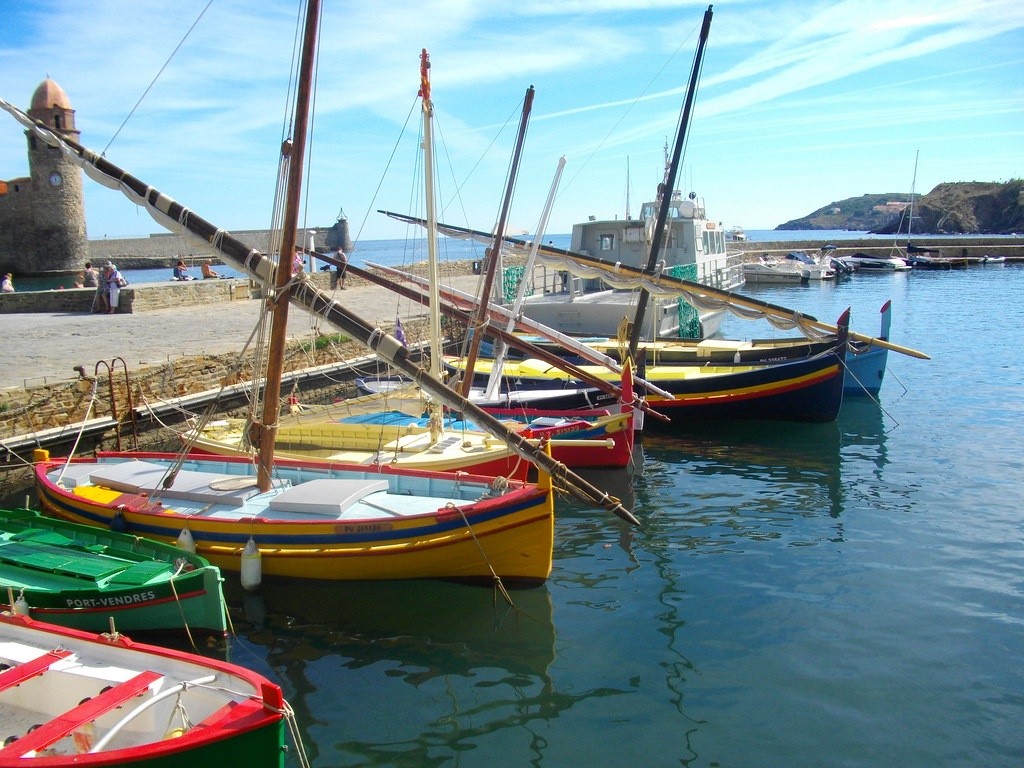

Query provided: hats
[104,261,112,267]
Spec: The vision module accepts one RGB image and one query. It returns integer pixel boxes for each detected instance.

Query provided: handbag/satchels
[114,271,129,288]
[3,280,14,292]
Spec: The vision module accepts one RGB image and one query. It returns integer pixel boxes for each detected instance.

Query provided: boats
[0,494,228,636]
[0,588,292,768]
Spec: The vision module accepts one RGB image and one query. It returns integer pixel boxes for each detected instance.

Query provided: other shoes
[341,287,346,290]
[108,309,115,314]
[104,310,109,314]
[94,310,101,313]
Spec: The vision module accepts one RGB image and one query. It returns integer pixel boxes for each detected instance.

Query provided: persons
[293,252,303,274]
[173,261,188,280]
[83,262,97,287]
[74,282,81,288]
[201,258,220,278]
[333,247,347,290]
[549,241,553,247]
[2,275,14,293]
[94,261,123,314]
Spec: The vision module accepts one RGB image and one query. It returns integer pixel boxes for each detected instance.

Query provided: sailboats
[8,1,558,585]
[338,5,1006,467]
